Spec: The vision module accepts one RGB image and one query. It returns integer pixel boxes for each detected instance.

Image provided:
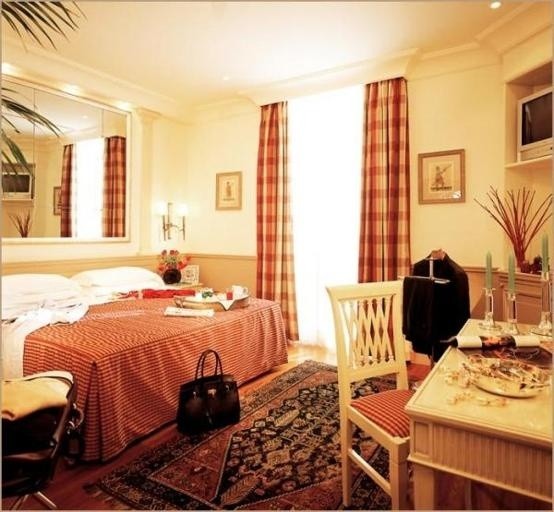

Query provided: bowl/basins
[463,357,550,399]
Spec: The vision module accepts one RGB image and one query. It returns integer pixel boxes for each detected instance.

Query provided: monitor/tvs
[517,86,554,161]
[2,172,33,200]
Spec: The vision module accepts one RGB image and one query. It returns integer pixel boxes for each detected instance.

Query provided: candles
[542,231,549,275]
[486,251,493,291]
[507,252,516,294]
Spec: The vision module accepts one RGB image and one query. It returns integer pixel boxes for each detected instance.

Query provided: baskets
[173,286,250,312]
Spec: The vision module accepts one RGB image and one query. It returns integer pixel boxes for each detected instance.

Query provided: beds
[1,265,289,467]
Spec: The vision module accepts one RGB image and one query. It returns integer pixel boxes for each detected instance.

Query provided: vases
[163,268,181,284]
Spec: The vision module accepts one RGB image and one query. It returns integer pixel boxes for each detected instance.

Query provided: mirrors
[1,70,134,244]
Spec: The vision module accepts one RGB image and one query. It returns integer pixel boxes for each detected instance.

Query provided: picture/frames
[214,171,242,210]
[415,148,467,206]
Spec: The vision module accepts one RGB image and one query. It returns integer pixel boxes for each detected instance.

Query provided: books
[439,334,553,369]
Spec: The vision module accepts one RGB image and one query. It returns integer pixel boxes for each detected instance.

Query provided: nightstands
[178,282,203,291]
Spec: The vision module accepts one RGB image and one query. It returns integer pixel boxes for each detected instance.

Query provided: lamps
[154,199,188,241]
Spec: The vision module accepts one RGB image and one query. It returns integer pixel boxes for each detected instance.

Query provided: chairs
[322,278,417,511]
[2,370,88,510]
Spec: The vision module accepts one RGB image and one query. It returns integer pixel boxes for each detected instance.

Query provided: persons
[403,249,471,363]
[436,166,444,190]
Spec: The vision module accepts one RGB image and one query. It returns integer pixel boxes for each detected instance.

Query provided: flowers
[156,249,191,274]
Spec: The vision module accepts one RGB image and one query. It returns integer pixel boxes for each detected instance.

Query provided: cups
[232,286,248,298]
[225,288,234,302]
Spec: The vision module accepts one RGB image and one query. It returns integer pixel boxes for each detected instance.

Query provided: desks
[404,313,553,511]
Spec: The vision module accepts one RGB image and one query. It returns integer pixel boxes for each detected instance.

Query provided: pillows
[69,266,166,290]
[1,274,83,314]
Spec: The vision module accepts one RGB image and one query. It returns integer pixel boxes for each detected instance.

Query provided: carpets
[81,357,416,510]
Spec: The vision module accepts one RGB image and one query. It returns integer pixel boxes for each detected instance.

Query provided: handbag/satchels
[176,350,242,437]
